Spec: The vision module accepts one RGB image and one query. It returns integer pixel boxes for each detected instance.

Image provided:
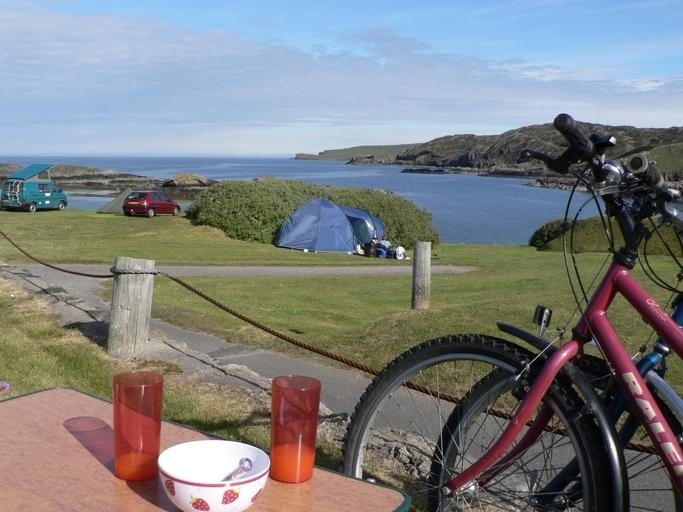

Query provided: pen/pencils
[2,163,68,212]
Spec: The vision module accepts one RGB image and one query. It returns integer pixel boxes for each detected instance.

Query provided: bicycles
[341,113,683,509]
[426,270,682,508]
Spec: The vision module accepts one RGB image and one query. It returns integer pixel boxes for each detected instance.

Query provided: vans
[2,163,68,212]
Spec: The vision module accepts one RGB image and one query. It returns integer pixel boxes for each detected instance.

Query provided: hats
[122,190,181,218]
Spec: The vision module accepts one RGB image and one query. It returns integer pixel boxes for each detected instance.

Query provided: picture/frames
[0,387,414,512]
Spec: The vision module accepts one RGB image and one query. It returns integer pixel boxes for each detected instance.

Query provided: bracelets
[157,440,271,512]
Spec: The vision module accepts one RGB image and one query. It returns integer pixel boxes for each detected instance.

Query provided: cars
[269,374,321,483]
[122,190,181,218]
[112,372,163,482]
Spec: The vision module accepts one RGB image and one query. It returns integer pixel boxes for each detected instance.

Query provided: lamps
[376,243,390,258]
